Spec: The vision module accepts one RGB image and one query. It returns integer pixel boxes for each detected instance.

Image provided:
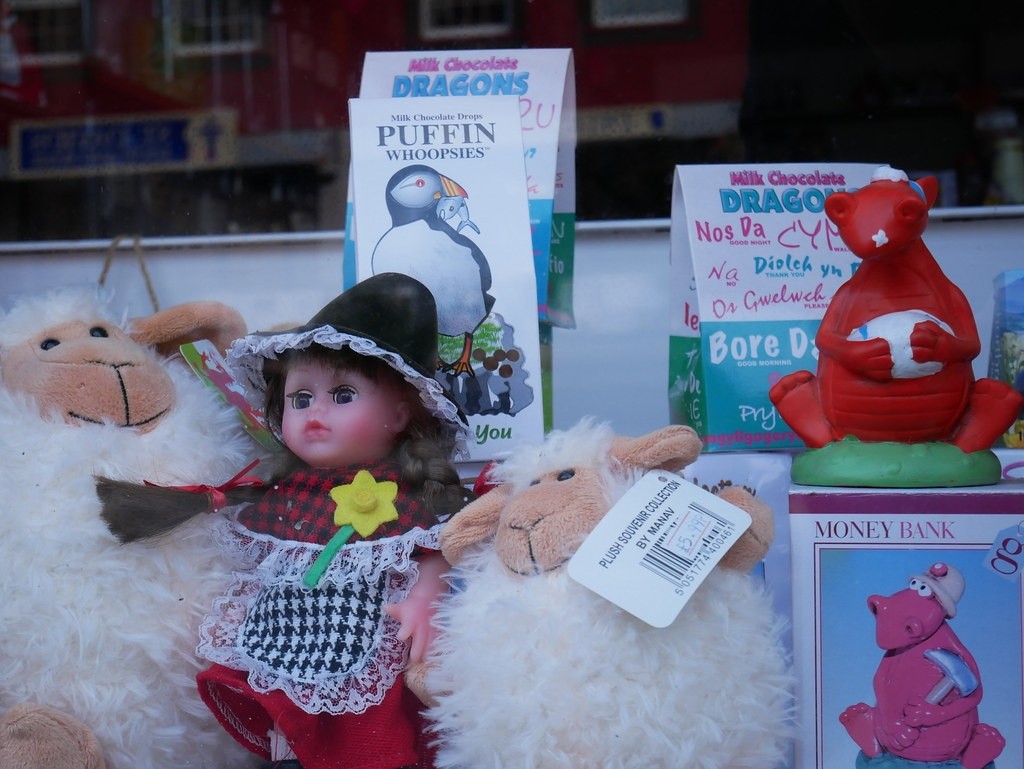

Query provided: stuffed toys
[0,290,287,769]
[420,417,798,769]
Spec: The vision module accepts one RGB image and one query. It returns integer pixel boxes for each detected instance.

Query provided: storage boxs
[787,478,1024,769]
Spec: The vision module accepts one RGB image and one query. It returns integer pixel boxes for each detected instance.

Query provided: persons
[95,273,479,769]
